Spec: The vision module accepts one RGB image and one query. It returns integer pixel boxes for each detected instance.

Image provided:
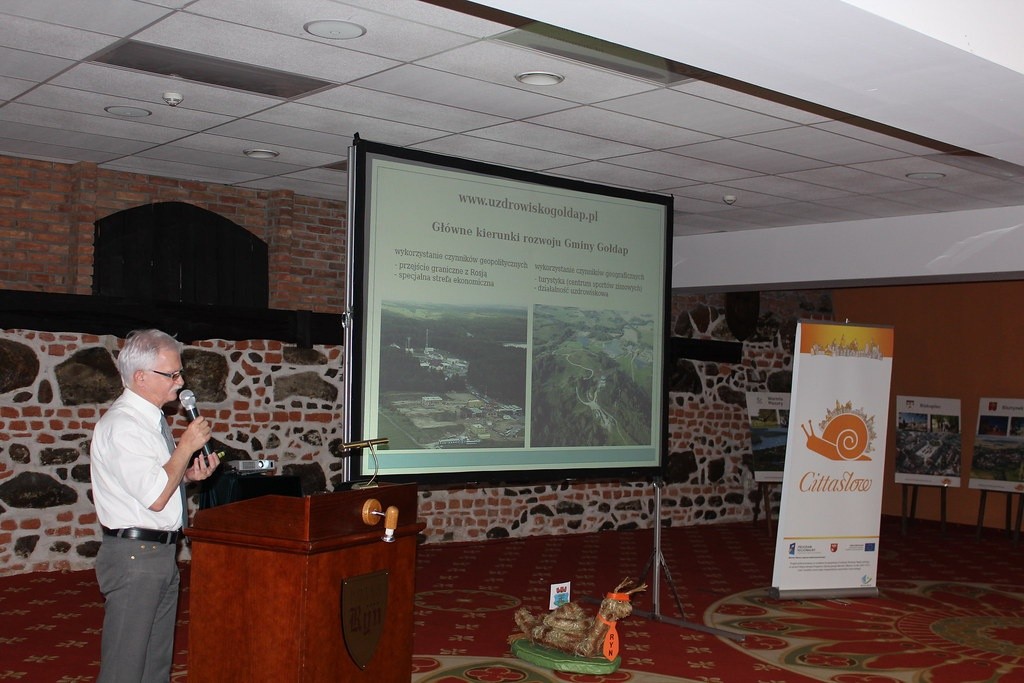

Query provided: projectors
[228,460,275,476]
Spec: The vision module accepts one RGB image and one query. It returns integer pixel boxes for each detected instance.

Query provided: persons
[90,330,220,683]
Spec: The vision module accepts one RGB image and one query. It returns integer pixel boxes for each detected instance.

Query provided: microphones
[179,390,211,457]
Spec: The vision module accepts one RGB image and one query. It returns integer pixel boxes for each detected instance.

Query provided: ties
[161,412,188,528]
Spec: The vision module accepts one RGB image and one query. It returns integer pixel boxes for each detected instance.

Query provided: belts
[102,526,178,544]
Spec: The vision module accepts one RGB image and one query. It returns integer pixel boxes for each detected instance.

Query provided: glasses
[148,368,183,381]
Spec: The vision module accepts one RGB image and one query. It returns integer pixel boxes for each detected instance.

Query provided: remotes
[204,449,226,467]
